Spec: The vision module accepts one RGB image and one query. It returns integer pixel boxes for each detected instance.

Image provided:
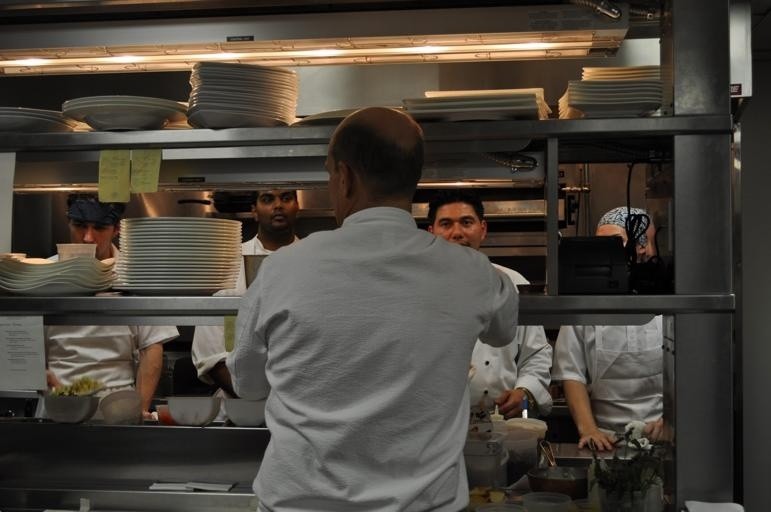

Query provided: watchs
[517,387,537,412]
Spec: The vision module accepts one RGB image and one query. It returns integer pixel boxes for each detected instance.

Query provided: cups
[464,391,621,511]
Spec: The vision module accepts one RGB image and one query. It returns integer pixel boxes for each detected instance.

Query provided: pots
[528,440,590,496]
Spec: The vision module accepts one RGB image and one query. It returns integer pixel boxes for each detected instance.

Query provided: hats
[65,195,119,227]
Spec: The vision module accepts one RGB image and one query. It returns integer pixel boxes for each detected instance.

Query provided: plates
[402,63,668,121]
[0,63,299,133]
[1,215,244,294]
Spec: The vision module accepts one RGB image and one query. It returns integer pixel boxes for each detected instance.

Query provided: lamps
[0,30,595,78]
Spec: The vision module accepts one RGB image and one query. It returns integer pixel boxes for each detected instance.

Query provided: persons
[228,104,520,511]
[555,207,679,450]
[191,186,301,398]
[40,190,180,418]
[425,195,554,428]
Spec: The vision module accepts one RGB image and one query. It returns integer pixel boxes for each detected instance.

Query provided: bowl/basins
[45,396,99,422]
[101,390,145,426]
[157,405,176,424]
[169,397,221,426]
[224,398,266,427]
